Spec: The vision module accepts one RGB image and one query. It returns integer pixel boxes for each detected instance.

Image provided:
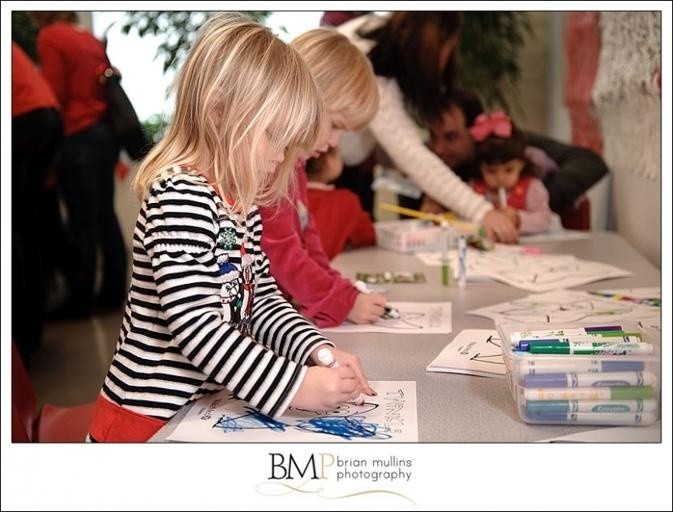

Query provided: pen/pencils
[495,244,545,253]
[509,326,660,424]
[355,280,401,319]
[499,189,507,212]
[318,348,365,406]
[458,233,466,290]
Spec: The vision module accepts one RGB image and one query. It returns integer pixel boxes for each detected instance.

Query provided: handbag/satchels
[95,63,155,161]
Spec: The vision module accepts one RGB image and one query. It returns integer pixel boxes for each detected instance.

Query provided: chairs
[12,334,96,443]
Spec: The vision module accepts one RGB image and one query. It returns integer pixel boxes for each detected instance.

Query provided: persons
[11,12,607,443]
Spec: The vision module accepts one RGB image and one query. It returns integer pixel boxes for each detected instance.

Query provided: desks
[146,229,660,444]
